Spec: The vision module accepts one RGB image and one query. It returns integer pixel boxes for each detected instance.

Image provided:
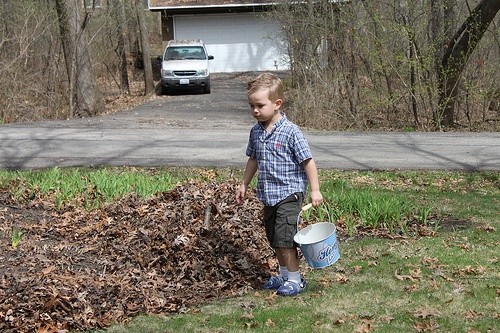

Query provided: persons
[236,72,323,296]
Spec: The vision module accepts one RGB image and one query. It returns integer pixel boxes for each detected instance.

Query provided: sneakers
[277,278,307,296]
[261,275,287,290]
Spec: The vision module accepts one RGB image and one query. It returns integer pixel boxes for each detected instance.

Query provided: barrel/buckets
[293,202,341,269]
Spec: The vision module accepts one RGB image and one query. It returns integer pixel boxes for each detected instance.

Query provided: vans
[156,38,214,93]
